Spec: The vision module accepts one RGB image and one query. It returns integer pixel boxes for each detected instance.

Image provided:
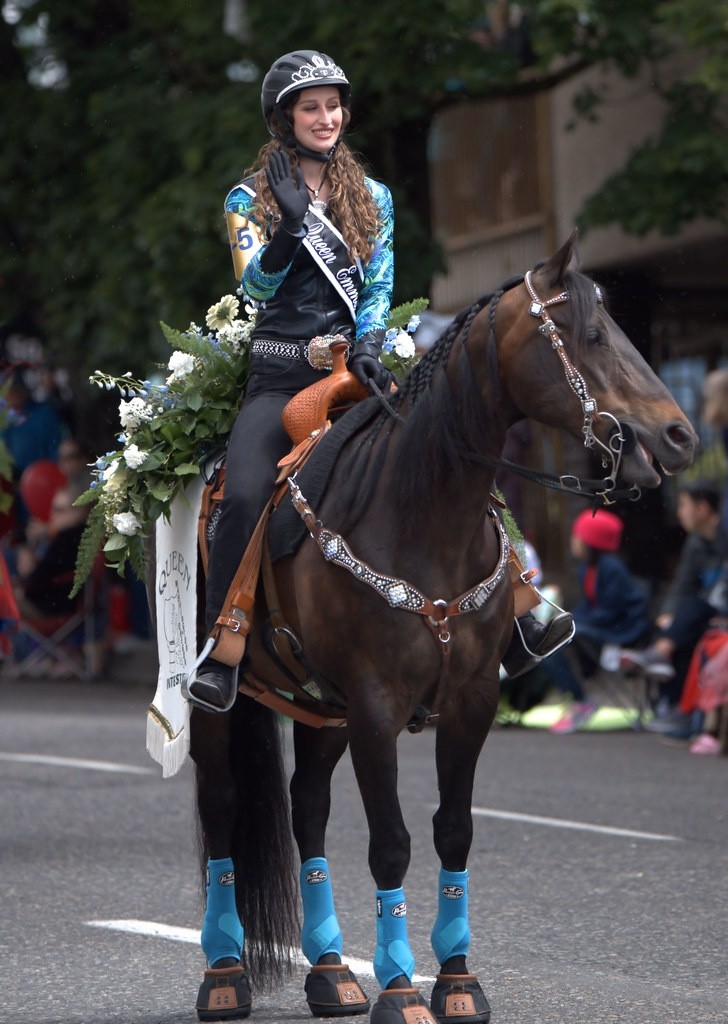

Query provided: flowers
[67,283,430,603]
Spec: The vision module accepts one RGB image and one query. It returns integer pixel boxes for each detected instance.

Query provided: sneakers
[549,699,601,736]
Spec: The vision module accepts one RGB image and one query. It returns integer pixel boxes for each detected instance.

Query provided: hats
[573,508,623,552]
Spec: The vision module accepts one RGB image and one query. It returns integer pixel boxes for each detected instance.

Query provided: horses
[145,227,698,1023]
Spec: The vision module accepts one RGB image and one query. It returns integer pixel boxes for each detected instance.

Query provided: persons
[182,49,578,709]
[0,329,726,758]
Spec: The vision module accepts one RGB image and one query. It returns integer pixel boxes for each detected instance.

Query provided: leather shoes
[501,610,573,678]
[185,670,230,716]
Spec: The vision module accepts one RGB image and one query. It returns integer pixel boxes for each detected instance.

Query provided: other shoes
[690,734,722,755]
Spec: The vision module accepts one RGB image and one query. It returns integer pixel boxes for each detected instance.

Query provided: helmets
[261,49,351,138]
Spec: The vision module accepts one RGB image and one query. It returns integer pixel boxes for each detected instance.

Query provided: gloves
[264,149,308,235]
[350,354,392,397]
